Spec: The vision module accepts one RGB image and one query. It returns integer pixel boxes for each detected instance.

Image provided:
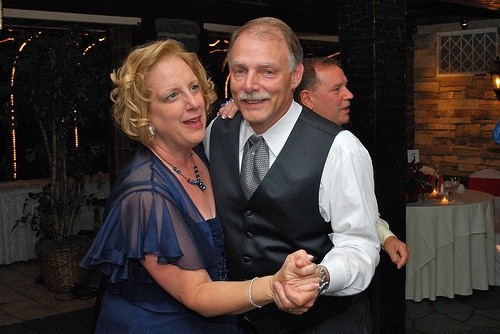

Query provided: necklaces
[152,146,206,191]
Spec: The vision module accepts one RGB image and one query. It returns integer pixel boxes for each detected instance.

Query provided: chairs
[468,169,500,218]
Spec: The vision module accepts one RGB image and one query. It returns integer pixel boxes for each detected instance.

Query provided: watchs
[317,265,329,294]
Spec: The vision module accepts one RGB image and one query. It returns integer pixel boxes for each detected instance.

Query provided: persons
[293,58,409,269]
[78,39,322,334]
[202,17,382,334]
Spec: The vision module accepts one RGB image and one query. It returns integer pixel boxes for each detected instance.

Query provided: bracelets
[249,277,261,308]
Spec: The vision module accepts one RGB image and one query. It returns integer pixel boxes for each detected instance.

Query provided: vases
[405,189,418,203]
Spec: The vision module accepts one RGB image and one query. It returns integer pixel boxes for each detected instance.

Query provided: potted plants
[0,28,113,294]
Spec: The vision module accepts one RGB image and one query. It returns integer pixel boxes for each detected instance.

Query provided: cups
[444,180,452,187]
[457,184,465,193]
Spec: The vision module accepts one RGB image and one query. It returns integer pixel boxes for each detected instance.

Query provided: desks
[405,189,500,302]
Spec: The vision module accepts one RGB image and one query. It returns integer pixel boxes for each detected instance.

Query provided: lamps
[460,14,468,27]
[486,55,500,102]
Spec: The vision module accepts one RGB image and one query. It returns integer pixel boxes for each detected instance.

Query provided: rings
[229,99,234,103]
[287,308,291,312]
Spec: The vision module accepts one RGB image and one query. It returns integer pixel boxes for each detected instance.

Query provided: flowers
[404,157,434,201]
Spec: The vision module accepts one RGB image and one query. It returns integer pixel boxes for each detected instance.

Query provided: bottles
[407,175,418,203]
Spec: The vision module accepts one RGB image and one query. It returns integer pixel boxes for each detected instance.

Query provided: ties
[240,135,270,200]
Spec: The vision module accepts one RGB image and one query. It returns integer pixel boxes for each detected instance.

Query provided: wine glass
[450,176,460,195]
